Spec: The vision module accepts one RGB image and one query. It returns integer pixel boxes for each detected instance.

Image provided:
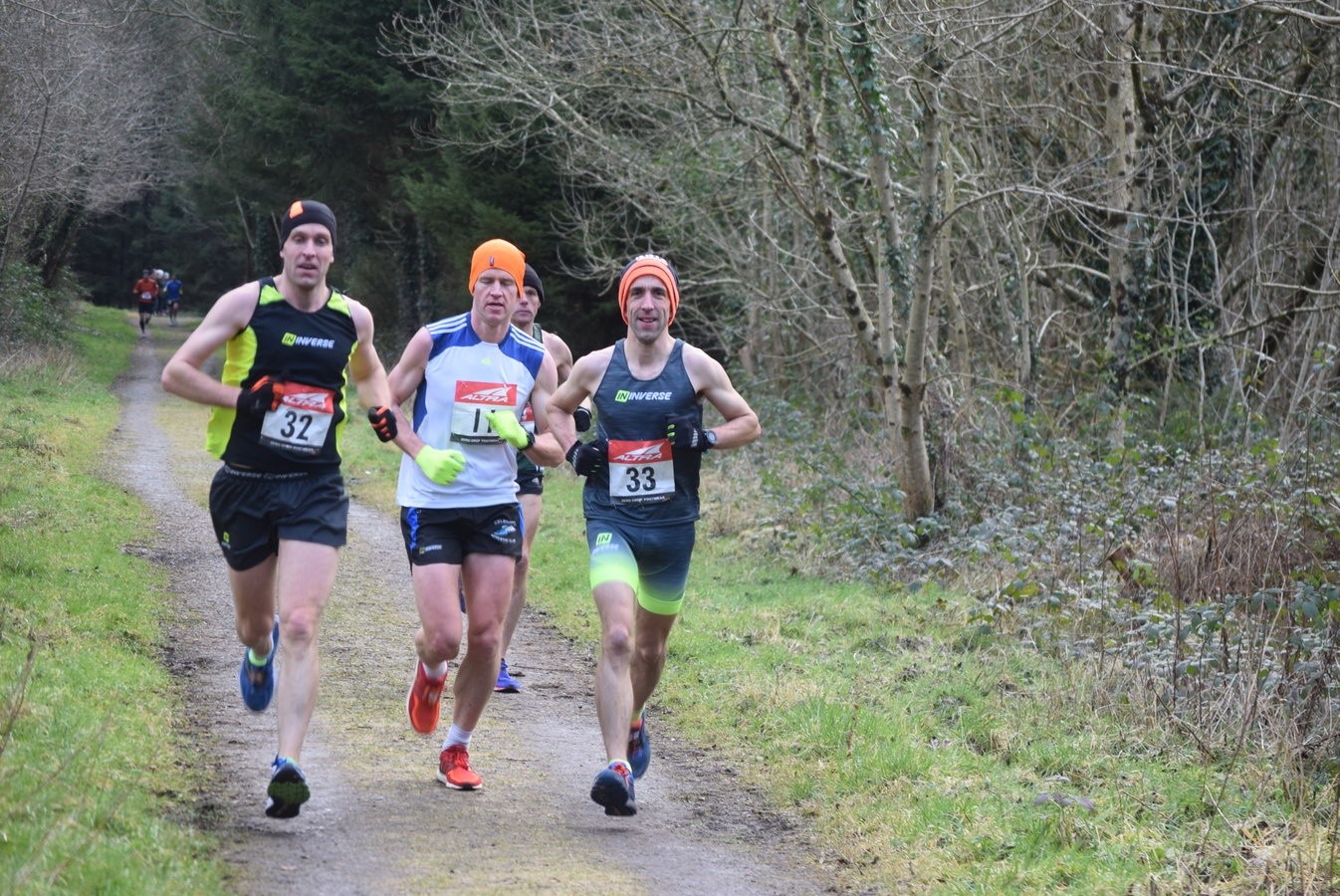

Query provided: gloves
[565,439,602,477]
[368,406,398,442]
[665,413,714,453]
[236,374,285,412]
[484,411,535,450]
[573,406,592,432]
[416,445,465,485]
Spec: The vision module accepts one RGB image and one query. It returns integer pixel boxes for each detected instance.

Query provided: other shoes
[142,331,145,337]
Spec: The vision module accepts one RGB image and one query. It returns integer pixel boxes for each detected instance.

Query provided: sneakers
[265,754,310,818]
[406,660,448,735]
[590,762,637,817]
[493,660,521,692]
[627,710,651,779]
[240,614,280,712]
[436,744,483,789]
[460,584,468,614]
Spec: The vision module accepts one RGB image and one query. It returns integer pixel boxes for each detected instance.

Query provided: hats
[280,199,337,250]
[522,263,545,305]
[468,239,526,300]
[619,254,679,326]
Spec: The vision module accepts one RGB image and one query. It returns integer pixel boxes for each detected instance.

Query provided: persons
[387,238,567,791]
[159,199,398,820]
[132,268,183,338]
[457,264,593,694]
[543,254,761,817]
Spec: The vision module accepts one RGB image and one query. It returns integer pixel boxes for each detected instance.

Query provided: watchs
[518,431,535,451]
[703,428,717,455]
